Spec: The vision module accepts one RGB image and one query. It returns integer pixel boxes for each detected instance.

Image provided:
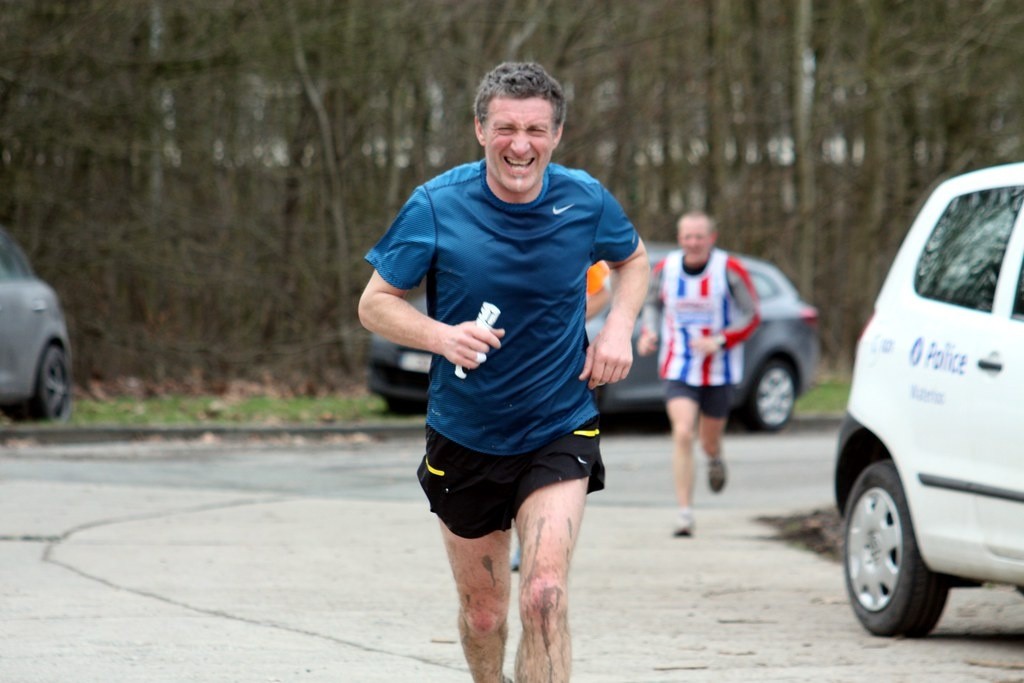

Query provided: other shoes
[710,454,725,492]
[674,517,691,536]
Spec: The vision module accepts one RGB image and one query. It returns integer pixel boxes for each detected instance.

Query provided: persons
[356,63,648,683]
[636,211,763,537]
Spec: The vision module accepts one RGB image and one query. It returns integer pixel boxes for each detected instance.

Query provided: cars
[833,163,1024,642]
[0,224,76,426]
[365,240,826,435]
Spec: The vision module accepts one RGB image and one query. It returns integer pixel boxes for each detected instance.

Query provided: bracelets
[713,334,726,348]
